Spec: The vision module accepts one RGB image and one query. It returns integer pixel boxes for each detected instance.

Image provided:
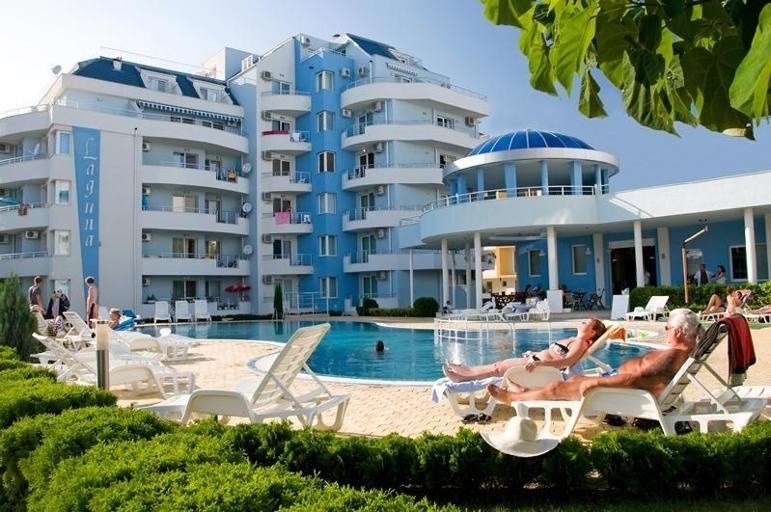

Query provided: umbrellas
[224,284,251,296]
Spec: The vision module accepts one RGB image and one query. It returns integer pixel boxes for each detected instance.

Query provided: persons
[442,317,606,384]
[748,304,771,314]
[446,300,453,313]
[561,284,570,304]
[525,284,533,296]
[108,307,122,328]
[486,307,701,405]
[28,276,42,312]
[533,283,544,298]
[694,263,743,317]
[84,275,97,327]
[376,340,385,352]
[56,289,71,319]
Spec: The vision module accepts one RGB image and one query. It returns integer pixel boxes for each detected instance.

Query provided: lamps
[585,247,592,255]
[539,249,546,256]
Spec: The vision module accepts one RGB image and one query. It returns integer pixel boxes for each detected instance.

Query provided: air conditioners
[338,66,387,281]
[0,188,10,197]
[302,36,311,45]
[142,140,151,288]
[262,71,272,285]
[0,230,38,244]
[466,115,476,126]
[0,143,11,154]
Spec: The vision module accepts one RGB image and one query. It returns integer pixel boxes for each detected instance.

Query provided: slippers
[478,413,491,423]
[462,413,477,422]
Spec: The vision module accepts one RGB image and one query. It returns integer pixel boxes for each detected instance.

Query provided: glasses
[665,326,675,330]
